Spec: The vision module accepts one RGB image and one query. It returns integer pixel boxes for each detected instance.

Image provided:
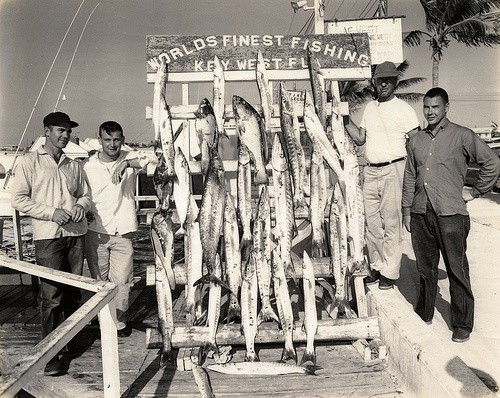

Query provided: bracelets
[124,159,130,167]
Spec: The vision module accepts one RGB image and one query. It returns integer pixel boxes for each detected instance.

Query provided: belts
[367,157,405,167]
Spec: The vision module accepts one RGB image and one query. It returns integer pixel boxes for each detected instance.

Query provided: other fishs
[154,252,176,357]
[192,148,228,290]
[330,182,356,316]
[308,140,329,257]
[198,253,224,355]
[271,133,297,280]
[235,141,258,256]
[158,58,347,255]
[253,186,280,328]
[301,249,320,368]
[269,251,298,364]
[239,253,261,363]
[206,364,323,377]
[344,137,374,283]
[174,147,192,238]
[222,190,244,323]
[190,363,215,397]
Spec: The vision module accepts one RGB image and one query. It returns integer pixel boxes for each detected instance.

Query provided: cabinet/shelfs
[145,32,382,371]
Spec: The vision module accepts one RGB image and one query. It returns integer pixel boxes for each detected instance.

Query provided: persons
[79,120,158,338]
[344,60,421,290]
[10,111,93,376]
[401,87,500,343]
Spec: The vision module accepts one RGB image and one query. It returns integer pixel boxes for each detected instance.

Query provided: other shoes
[117,309,127,331]
[363,269,381,285]
[379,274,395,289]
[452,326,470,342]
[44,355,61,374]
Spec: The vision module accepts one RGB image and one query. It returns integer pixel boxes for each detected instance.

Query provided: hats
[373,61,399,78]
[43,112,79,128]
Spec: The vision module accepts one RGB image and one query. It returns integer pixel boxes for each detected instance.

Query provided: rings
[60,220,64,224]
